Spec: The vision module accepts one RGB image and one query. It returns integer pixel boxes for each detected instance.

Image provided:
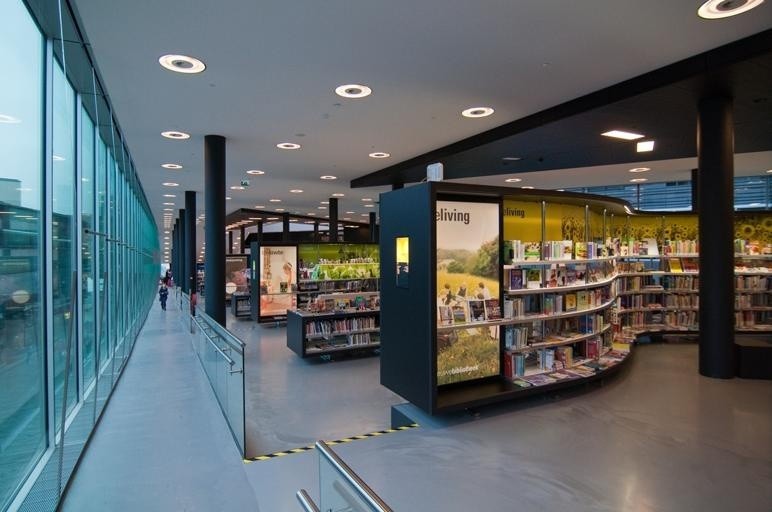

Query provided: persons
[158,282,169,310]
[283,261,296,293]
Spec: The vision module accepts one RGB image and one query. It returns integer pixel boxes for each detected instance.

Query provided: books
[734,240,771,328]
[620,238,699,328]
[503,239,621,378]
[298,277,380,351]
